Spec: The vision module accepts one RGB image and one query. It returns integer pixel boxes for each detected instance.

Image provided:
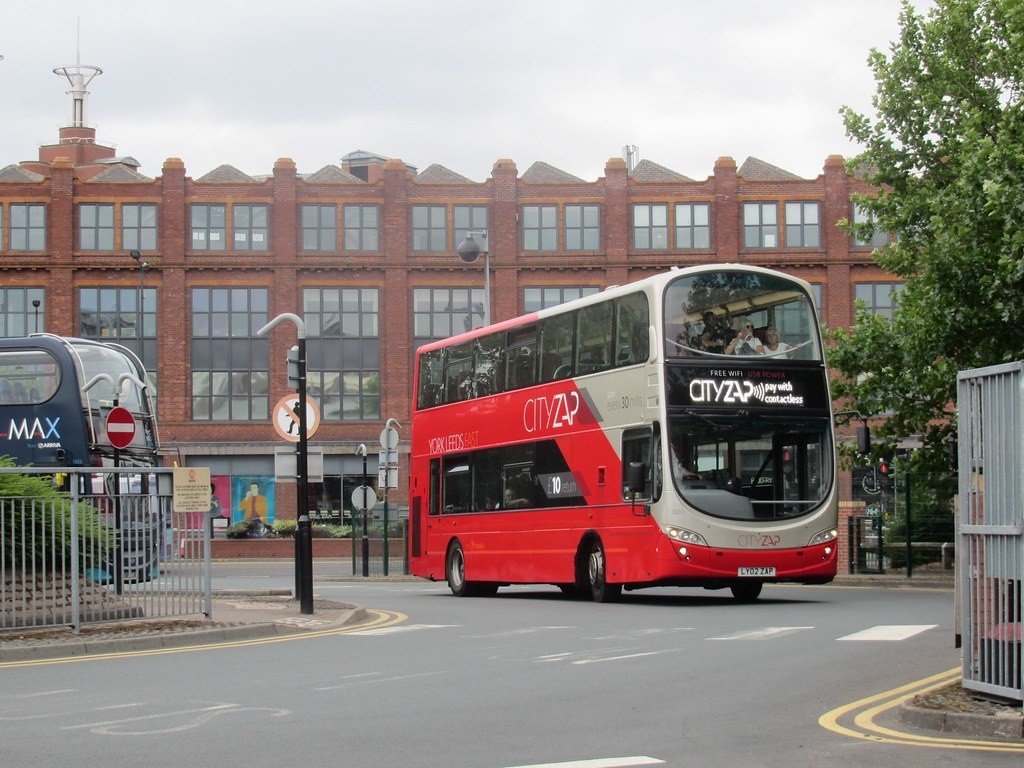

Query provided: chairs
[0,377,41,404]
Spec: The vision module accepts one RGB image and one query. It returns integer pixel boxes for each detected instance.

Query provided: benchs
[423,322,770,406]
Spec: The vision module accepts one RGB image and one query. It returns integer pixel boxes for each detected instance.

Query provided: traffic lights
[877,460,888,485]
[782,445,794,473]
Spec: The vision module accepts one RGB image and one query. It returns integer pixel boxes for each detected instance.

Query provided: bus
[409,263,870,603]
[0,331,182,584]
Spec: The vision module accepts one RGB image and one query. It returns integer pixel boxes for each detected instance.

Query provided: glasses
[744,325,754,329]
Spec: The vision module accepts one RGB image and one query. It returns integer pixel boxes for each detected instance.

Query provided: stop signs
[105,407,136,449]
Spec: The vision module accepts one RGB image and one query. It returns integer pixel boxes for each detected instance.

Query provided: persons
[496,487,531,508]
[700,312,727,352]
[724,319,762,356]
[756,327,795,360]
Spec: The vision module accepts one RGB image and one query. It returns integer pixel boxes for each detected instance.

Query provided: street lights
[130,249,148,368]
[32,300,41,333]
[457,230,491,328]
